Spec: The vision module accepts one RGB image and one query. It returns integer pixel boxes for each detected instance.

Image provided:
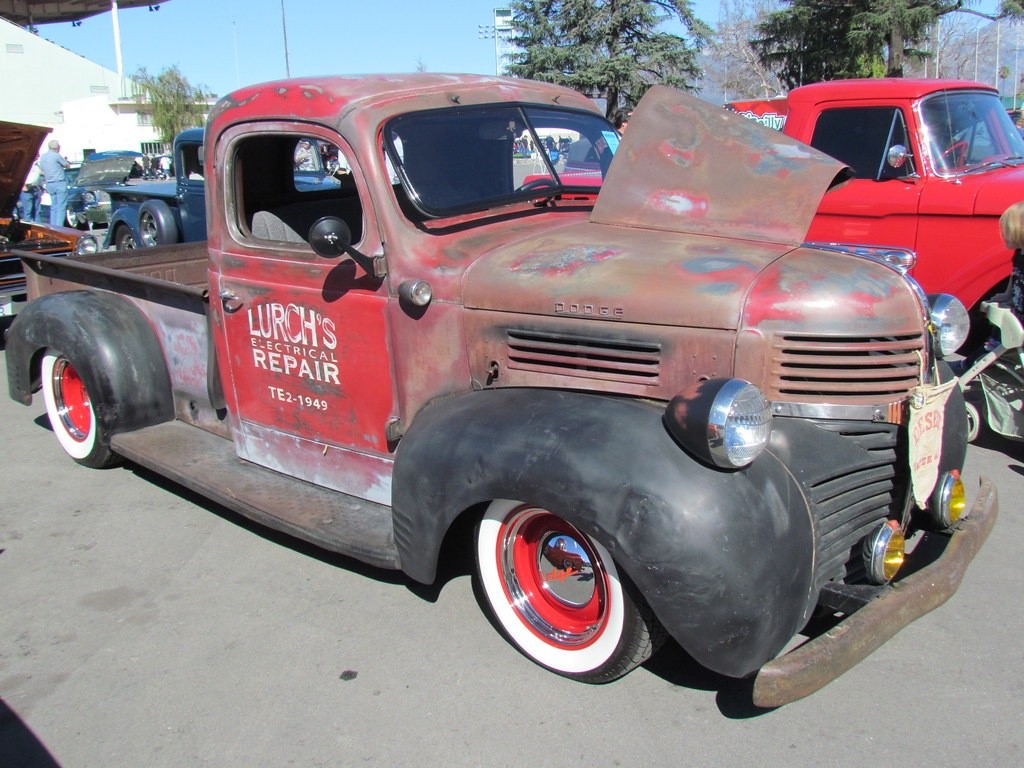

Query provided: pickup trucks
[14,149,144,228]
[0,119,102,351]
[104,128,342,250]
[4,72,1000,708]
[524,78,1024,358]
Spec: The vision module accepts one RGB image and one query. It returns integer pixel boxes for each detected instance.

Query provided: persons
[40,140,71,226]
[609,106,633,141]
[20,151,43,223]
[159,154,172,180]
[319,146,340,175]
[1015,119,1024,140]
[295,139,315,171]
[545,134,555,150]
[142,154,157,172]
[514,136,533,158]
[559,135,572,151]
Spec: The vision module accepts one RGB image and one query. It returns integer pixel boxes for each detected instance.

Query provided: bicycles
[959,298,1024,442]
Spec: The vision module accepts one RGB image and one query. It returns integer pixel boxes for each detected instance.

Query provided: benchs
[251,186,437,247]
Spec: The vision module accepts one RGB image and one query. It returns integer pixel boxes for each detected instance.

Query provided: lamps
[72,20,81,27]
[148,5,160,13]
